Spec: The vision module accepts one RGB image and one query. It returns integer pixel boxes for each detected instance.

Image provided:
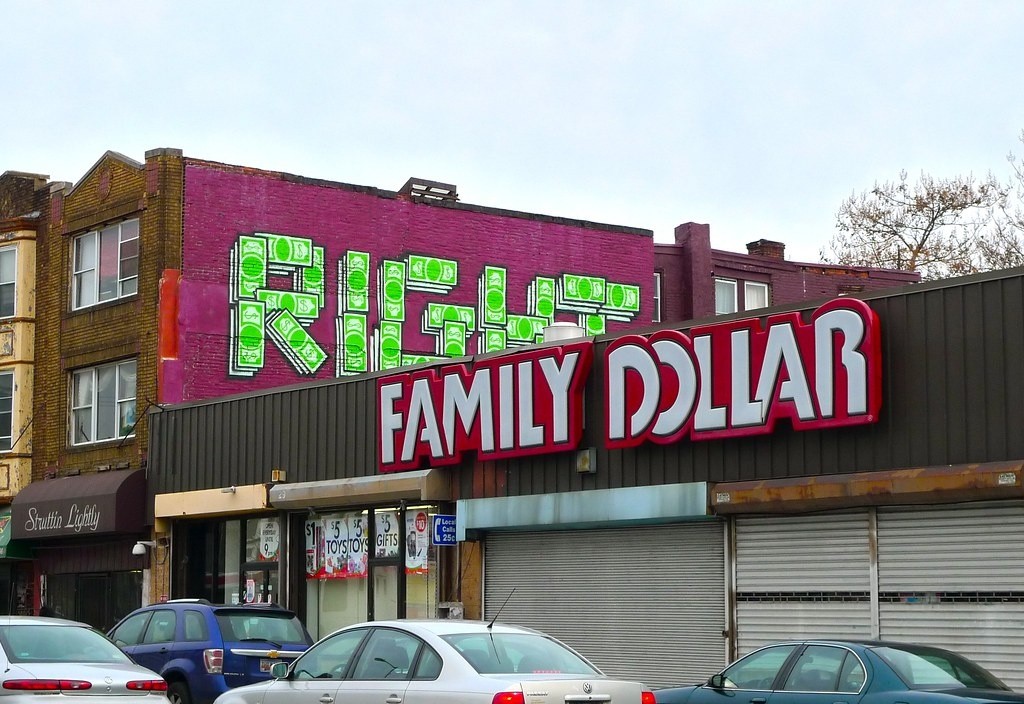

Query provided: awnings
[11,468,145,541]
[0,512,38,561]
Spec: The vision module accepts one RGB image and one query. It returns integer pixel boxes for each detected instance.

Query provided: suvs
[105,598,314,704]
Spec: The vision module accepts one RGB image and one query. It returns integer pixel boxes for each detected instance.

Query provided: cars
[0,615,173,704]
[212,620,656,704]
[652,641,1024,704]
